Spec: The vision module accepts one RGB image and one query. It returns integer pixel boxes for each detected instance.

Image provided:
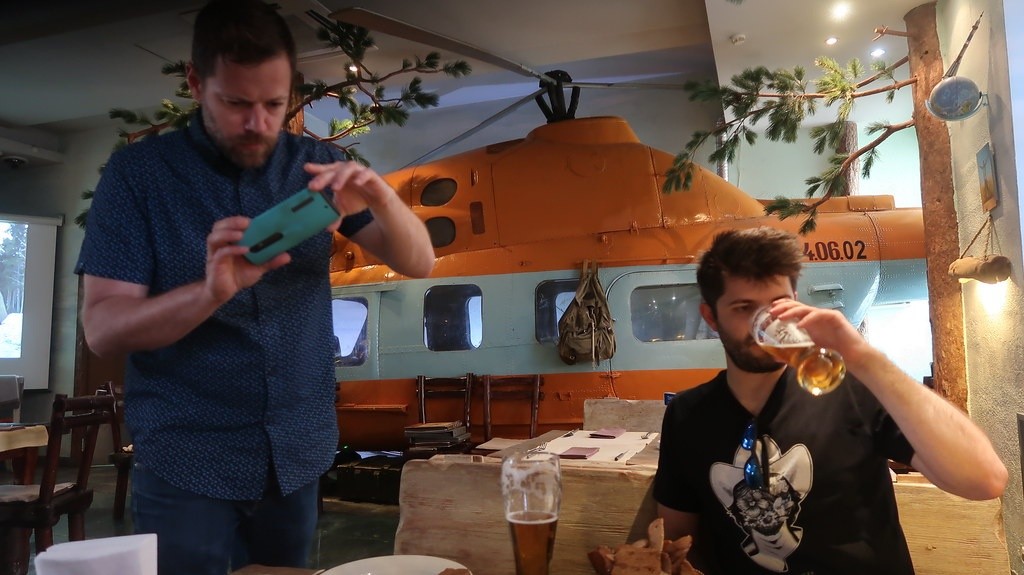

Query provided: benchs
[394,460,1010,575]
[583,397,667,434]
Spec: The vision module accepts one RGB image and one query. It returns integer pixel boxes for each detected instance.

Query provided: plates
[317,554,473,575]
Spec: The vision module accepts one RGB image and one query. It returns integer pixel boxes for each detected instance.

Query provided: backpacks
[559,258,613,364]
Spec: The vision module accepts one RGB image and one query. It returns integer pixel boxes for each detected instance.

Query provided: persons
[653,230,1009,574]
[73,1,436,575]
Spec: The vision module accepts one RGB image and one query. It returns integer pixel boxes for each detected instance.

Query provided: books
[588,427,626,439]
[404,419,471,457]
[558,446,600,459]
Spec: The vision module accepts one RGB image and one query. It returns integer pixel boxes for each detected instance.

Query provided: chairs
[409,373,476,459]
[100,382,134,520]
[470,375,544,456]
[0,389,114,575]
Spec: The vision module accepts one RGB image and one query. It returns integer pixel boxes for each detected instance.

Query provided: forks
[528,441,548,457]
[641,432,653,439]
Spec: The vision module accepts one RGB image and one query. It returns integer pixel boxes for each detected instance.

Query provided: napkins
[559,447,599,459]
[589,428,626,439]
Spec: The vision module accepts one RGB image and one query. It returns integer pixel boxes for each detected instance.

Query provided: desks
[0,424,49,485]
[486,430,661,469]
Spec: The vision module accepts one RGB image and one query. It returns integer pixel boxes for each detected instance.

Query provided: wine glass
[747,304,846,397]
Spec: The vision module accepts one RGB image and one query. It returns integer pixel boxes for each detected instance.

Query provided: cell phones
[233,188,341,266]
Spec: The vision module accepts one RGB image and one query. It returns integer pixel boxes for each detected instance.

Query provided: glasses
[742,420,770,491]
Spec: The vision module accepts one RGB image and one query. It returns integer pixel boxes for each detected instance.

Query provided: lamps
[948,217,1011,284]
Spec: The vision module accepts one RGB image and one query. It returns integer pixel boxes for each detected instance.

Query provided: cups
[501,453,562,575]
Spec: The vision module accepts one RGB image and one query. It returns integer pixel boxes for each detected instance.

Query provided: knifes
[615,451,628,461]
[564,428,579,437]
